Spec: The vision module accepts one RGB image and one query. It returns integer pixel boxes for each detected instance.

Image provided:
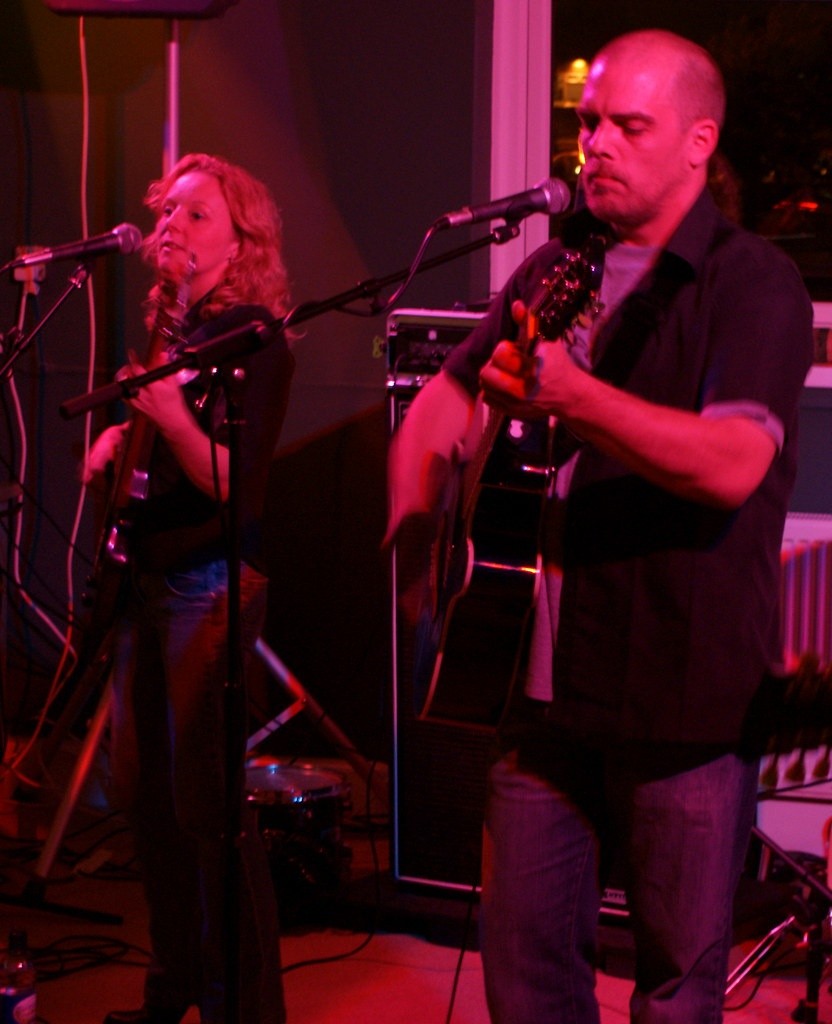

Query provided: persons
[81,151,295,1024]
[379,28,815,1024]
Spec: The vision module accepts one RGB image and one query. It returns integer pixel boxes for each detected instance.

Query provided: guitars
[73,251,197,644]
[413,235,607,738]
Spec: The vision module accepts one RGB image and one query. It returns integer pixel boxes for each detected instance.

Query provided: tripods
[18,20,384,907]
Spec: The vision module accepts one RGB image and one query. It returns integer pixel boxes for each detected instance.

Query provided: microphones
[12,223,142,267]
[433,178,571,232]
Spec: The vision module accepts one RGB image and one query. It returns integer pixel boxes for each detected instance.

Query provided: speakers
[383,391,638,921]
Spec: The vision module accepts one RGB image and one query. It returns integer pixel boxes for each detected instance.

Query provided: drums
[244,764,344,928]
